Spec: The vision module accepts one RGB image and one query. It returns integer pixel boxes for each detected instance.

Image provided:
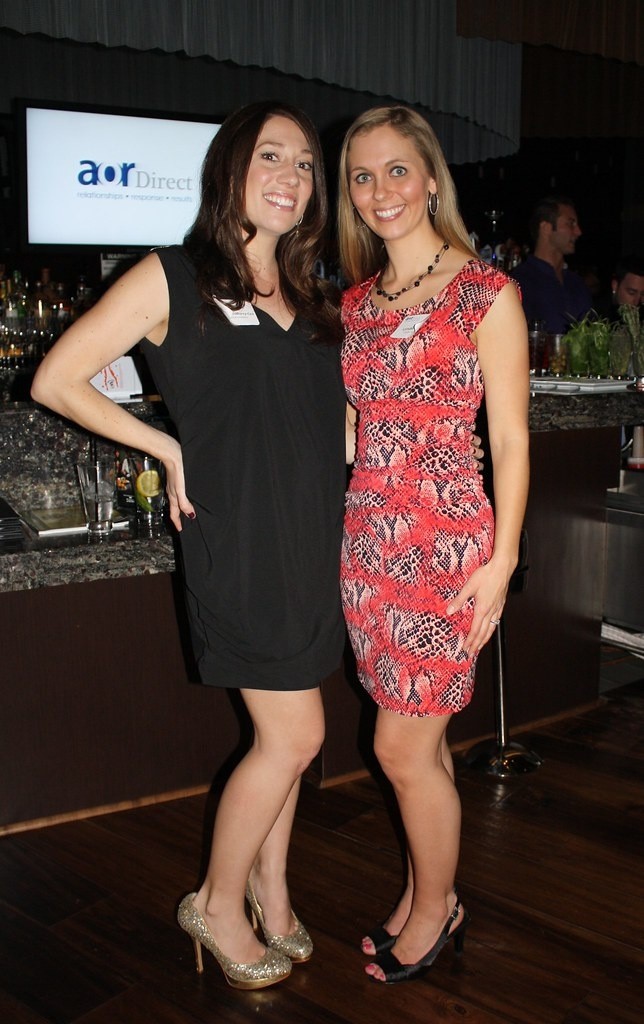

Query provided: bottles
[0,264,93,358]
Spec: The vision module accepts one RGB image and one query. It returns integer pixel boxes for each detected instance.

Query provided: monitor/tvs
[14,97,228,251]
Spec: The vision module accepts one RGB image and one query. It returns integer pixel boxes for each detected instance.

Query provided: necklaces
[374,238,450,301]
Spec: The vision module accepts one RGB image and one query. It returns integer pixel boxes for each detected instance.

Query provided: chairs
[463,429,548,776]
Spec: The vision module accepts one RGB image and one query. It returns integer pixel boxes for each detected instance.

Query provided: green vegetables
[558,305,642,377]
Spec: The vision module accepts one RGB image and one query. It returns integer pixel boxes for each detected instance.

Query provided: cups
[526,329,633,381]
[76,459,117,537]
[126,456,167,527]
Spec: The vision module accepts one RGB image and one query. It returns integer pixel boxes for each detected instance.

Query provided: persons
[26,99,486,992]
[502,192,621,334]
[333,106,529,984]
[607,260,644,320]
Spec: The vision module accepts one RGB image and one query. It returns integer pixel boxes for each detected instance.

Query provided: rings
[490,617,501,626]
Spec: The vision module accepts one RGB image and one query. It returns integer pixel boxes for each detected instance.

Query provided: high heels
[361,926,402,956]
[244,874,313,963]
[179,895,291,990]
[369,895,467,983]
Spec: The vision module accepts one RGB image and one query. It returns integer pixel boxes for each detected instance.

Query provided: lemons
[136,470,160,511]
